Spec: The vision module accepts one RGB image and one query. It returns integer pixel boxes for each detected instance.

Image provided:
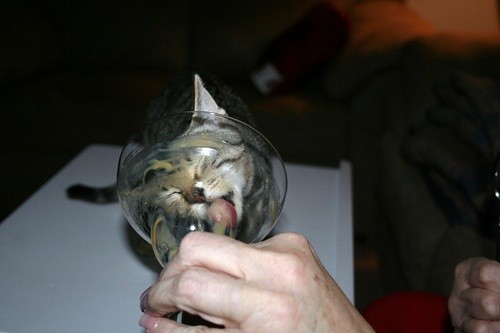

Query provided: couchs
[0,0,500,238]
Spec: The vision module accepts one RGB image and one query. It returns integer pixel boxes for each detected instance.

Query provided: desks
[0,143,354,333]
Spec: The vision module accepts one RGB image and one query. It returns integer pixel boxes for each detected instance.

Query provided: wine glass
[117,112,287,326]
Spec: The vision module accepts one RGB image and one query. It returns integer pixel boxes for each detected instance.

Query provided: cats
[65,74,288,329]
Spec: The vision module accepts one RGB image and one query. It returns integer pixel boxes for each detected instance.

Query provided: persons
[139,229,500,333]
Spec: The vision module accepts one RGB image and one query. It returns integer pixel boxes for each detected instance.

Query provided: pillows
[324,1,453,100]
